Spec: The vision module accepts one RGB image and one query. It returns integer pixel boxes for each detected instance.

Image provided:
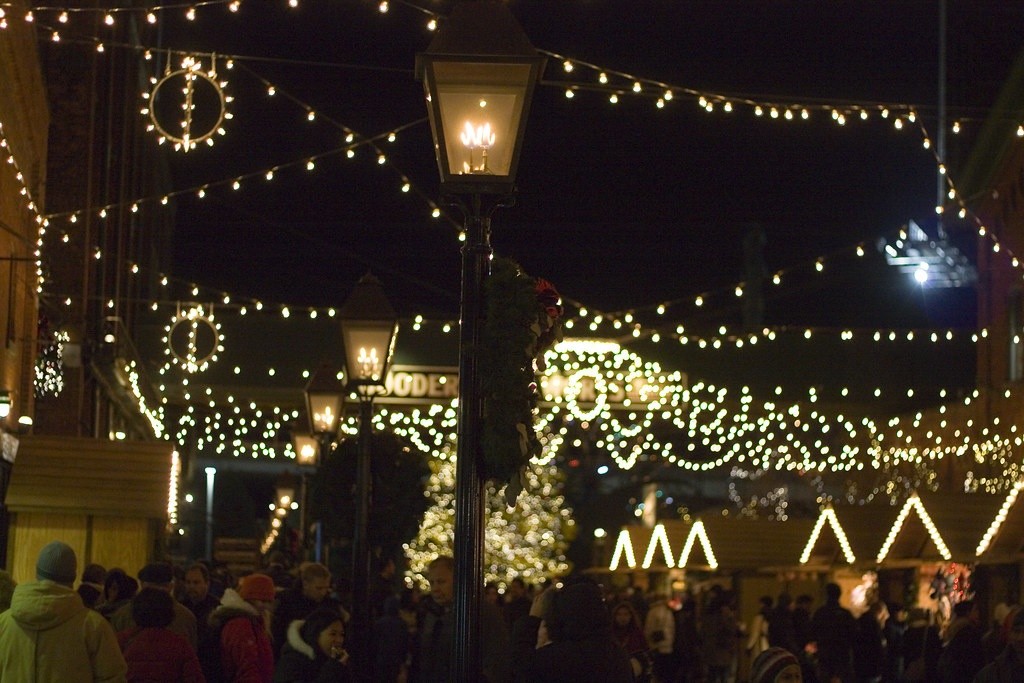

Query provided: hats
[241,575,275,603]
[35,542,77,584]
[546,583,610,640]
[752,647,800,683]
[138,560,174,583]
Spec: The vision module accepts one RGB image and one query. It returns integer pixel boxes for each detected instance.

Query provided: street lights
[339,271,399,682]
[416,1,551,683]
[302,361,348,561]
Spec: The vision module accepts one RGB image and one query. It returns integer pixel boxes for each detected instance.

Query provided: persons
[75,554,1024,683]
[533,278,562,371]
[0,540,128,683]
